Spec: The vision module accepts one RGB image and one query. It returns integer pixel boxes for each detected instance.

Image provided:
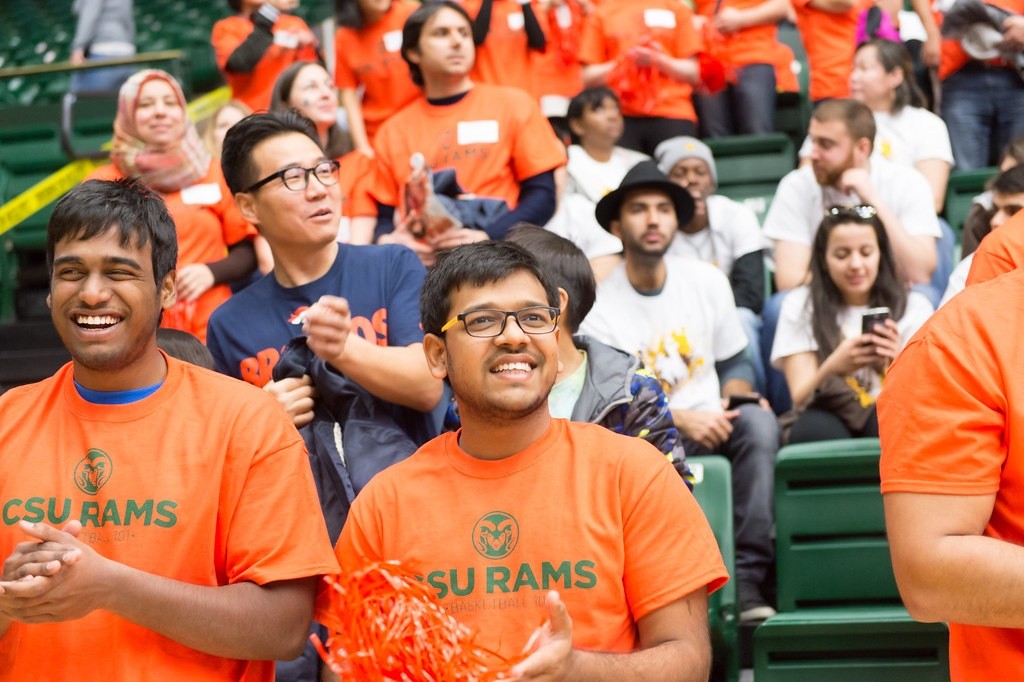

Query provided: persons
[63,0,1024,308]
[203,104,429,682]
[313,239,729,682]
[578,160,782,623]
[0,174,344,682]
[151,326,216,378]
[875,265,1024,682]
[440,221,695,495]
[768,205,933,434]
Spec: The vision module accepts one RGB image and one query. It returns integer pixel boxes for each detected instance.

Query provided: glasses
[824,204,879,220]
[242,159,340,195]
[438,306,559,337]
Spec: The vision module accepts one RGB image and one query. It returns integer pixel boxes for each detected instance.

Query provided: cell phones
[724,395,760,412]
[862,307,892,347]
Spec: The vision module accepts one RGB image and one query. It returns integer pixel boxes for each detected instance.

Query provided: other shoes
[739,602,775,620]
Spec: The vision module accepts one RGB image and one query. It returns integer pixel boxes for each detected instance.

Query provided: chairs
[0,0,1000,682]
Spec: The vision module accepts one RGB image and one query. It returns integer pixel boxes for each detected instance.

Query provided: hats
[594,161,696,234]
[652,135,716,183]
